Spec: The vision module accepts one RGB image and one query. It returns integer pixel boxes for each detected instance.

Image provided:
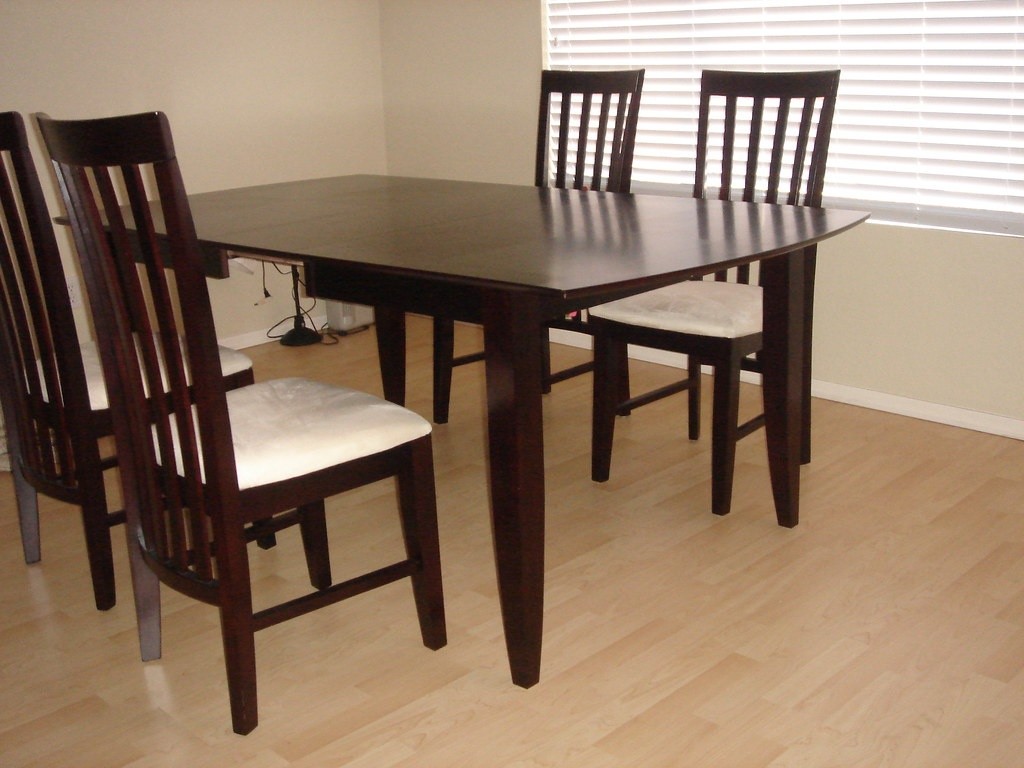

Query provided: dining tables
[54,175,873,691]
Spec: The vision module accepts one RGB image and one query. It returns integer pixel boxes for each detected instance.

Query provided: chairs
[0,109,278,612]
[586,68,842,530]
[32,110,447,736]
[421,67,646,429]
